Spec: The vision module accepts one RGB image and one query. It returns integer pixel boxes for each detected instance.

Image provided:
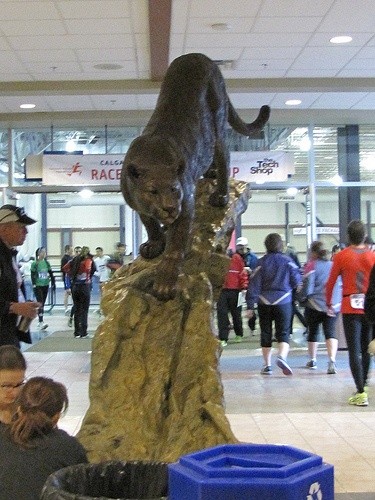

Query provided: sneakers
[348,385,369,405]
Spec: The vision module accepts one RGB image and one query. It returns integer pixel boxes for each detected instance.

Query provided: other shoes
[289,334,292,341]
[306,360,317,369]
[219,340,227,347]
[260,366,272,375]
[251,330,256,336]
[76,334,94,339]
[234,335,242,343]
[327,363,336,374]
[275,358,292,375]
[37,322,48,330]
[303,328,308,335]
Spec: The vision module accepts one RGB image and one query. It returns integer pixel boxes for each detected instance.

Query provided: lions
[121,52,270,301]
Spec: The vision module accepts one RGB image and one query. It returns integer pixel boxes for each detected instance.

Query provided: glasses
[0,376,29,394]
[0,207,25,223]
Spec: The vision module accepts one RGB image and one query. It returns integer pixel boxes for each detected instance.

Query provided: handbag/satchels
[63,260,73,274]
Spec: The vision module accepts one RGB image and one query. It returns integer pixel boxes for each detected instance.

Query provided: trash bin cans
[46,460,168,500]
[167,444,334,500]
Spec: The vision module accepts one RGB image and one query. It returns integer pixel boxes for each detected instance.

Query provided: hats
[0,205,36,225]
[236,237,248,245]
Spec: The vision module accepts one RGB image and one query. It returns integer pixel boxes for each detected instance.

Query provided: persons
[0,205,42,348]
[235,237,259,338]
[245,233,303,376]
[31,247,55,330]
[325,221,375,406]
[364,236,375,254]
[299,241,343,374]
[106,242,127,278]
[217,248,249,347]
[0,345,26,424]
[0,377,89,500]
[62,247,96,338]
[364,262,375,359]
[61,245,83,328]
[279,234,309,340]
[93,247,112,283]
